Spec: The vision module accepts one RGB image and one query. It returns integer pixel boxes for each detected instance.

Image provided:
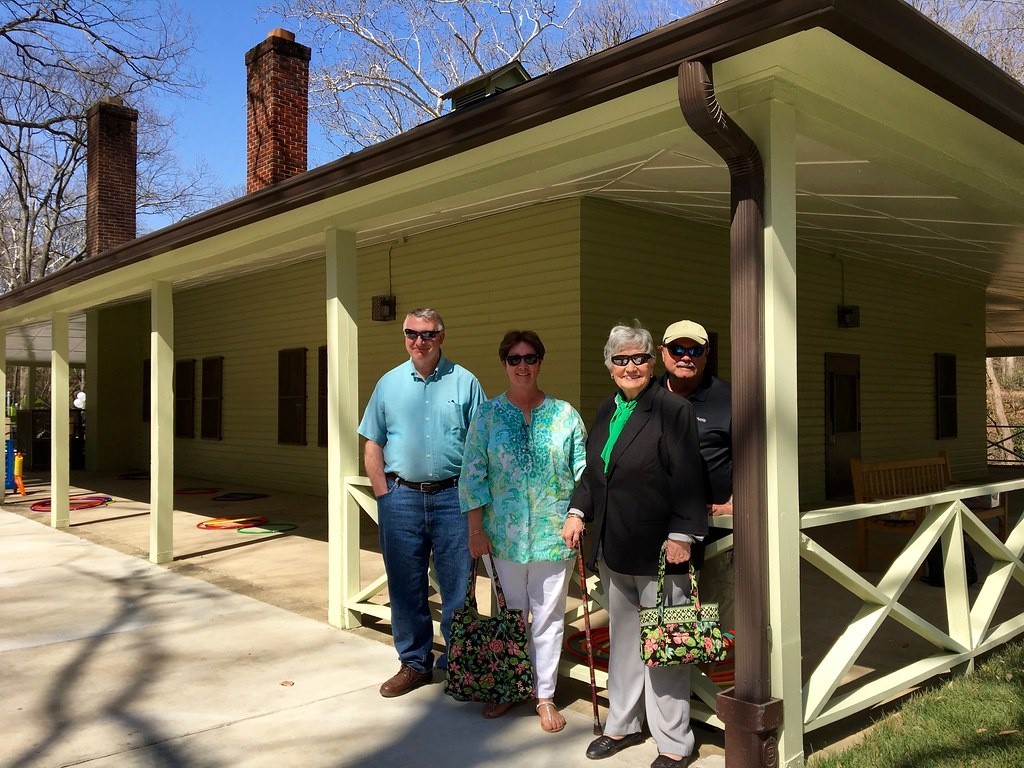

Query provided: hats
[662,319,709,345]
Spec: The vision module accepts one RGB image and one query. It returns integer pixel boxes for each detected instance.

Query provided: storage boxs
[948,482,1001,509]
[864,493,937,523]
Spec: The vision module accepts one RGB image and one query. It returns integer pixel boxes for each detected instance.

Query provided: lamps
[371,294,396,321]
[838,305,860,326]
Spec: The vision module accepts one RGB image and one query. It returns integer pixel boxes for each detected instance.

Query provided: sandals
[536,701,564,732]
[482,696,520,719]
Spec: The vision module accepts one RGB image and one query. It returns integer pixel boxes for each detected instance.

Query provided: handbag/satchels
[445,553,534,702]
[639,541,726,668]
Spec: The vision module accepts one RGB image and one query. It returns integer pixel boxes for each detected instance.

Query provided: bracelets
[566,513,581,519]
[469,529,480,538]
[728,497,733,506]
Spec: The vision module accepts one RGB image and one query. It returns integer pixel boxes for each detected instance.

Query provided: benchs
[848,457,1009,586]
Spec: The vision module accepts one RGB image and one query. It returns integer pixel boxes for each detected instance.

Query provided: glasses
[504,354,541,365]
[403,328,440,340]
[664,344,708,357]
[611,353,652,366]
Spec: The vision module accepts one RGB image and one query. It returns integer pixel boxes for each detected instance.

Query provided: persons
[561,325,709,768]
[657,320,734,634]
[458,330,588,734]
[357,308,488,697]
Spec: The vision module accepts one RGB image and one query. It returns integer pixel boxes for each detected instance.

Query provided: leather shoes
[651,744,697,768]
[586,731,641,759]
[380,665,432,697]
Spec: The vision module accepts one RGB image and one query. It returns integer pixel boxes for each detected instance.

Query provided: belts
[387,472,460,493]
